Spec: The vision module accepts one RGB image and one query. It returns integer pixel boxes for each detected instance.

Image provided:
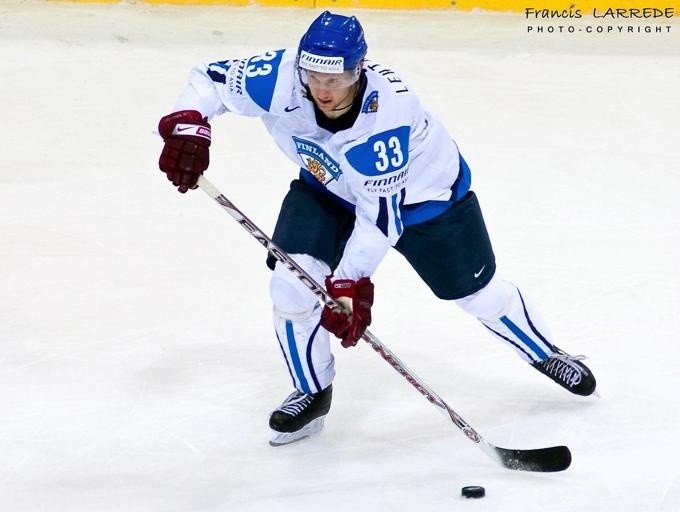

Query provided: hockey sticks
[197,176,570,471]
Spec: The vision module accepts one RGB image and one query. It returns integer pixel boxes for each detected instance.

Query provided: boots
[525,348,595,396]
[269,387,332,432]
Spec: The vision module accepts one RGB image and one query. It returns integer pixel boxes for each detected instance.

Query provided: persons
[157,8,597,432]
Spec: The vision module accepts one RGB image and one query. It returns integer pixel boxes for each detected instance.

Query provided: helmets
[295,12,367,89]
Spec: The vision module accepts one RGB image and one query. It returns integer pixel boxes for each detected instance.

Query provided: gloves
[159,110,211,193]
[321,274,373,348]
